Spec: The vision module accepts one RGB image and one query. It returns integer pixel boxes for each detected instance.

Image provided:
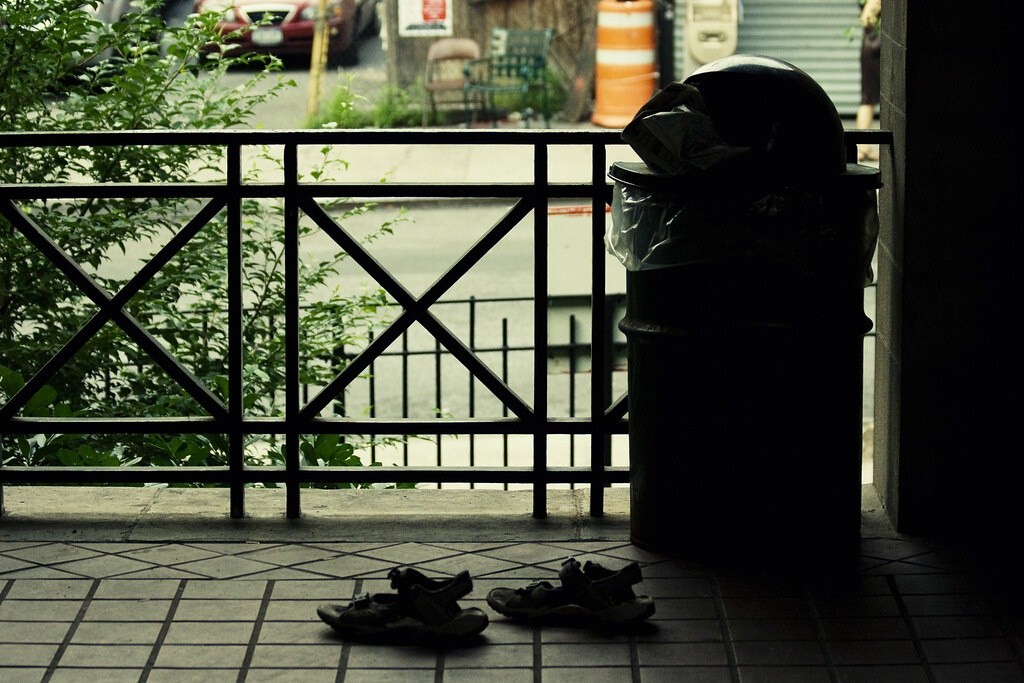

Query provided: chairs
[422,37,478,130]
[489,26,552,130]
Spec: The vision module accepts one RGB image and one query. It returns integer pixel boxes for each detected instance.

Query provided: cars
[191,1,384,71]
[12,0,163,94]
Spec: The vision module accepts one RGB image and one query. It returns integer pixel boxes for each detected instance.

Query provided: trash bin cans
[591,0,658,129]
[607,51,883,566]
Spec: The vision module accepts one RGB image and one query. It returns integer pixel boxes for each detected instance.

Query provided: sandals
[486,558,655,627]
[316,566,488,638]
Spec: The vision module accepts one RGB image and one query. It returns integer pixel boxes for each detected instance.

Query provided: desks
[463,53,550,129]
